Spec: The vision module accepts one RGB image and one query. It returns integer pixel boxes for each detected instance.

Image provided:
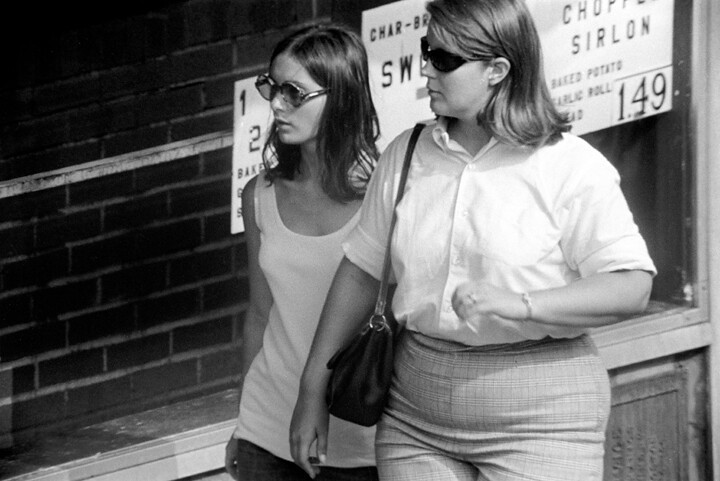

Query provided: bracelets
[520,292,533,319]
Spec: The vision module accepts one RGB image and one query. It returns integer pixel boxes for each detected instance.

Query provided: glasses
[420,36,478,73]
[255,73,328,109]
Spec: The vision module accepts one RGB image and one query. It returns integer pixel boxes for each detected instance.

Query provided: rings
[469,294,476,305]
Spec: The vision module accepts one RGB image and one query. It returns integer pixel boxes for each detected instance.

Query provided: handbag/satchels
[324,296,400,426]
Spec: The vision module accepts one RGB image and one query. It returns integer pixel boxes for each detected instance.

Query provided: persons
[288,0,658,481]
[222,25,382,481]
